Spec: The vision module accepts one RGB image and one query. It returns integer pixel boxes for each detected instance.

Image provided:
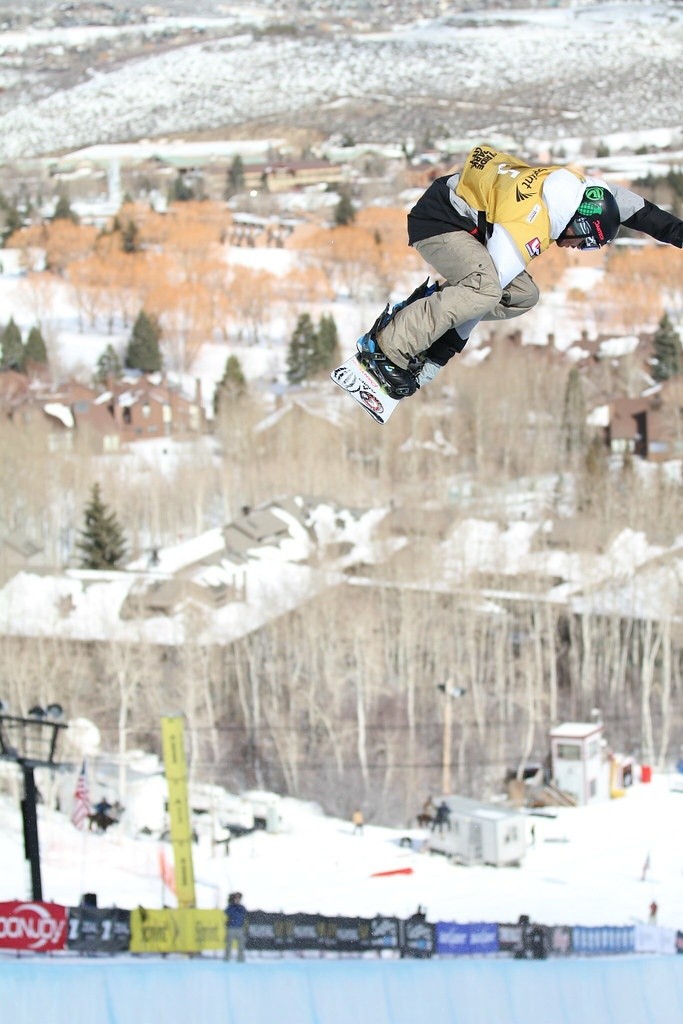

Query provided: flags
[72,767,90,830]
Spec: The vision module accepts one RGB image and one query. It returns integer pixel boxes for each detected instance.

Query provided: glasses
[582,243,600,250]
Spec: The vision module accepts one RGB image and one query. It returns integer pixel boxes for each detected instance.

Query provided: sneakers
[357,303,420,400]
[392,276,440,375]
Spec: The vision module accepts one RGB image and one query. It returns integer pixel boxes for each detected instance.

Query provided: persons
[423,796,451,831]
[96,799,110,816]
[357,146,683,396]
[225,893,246,961]
[353,809,364,833]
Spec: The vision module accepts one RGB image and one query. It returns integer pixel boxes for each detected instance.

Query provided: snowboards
[331,350,402,426]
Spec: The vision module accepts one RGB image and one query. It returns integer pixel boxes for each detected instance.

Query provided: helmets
[570,186,621,249]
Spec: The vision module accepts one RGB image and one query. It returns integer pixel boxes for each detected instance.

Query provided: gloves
[416,357,442,389]
